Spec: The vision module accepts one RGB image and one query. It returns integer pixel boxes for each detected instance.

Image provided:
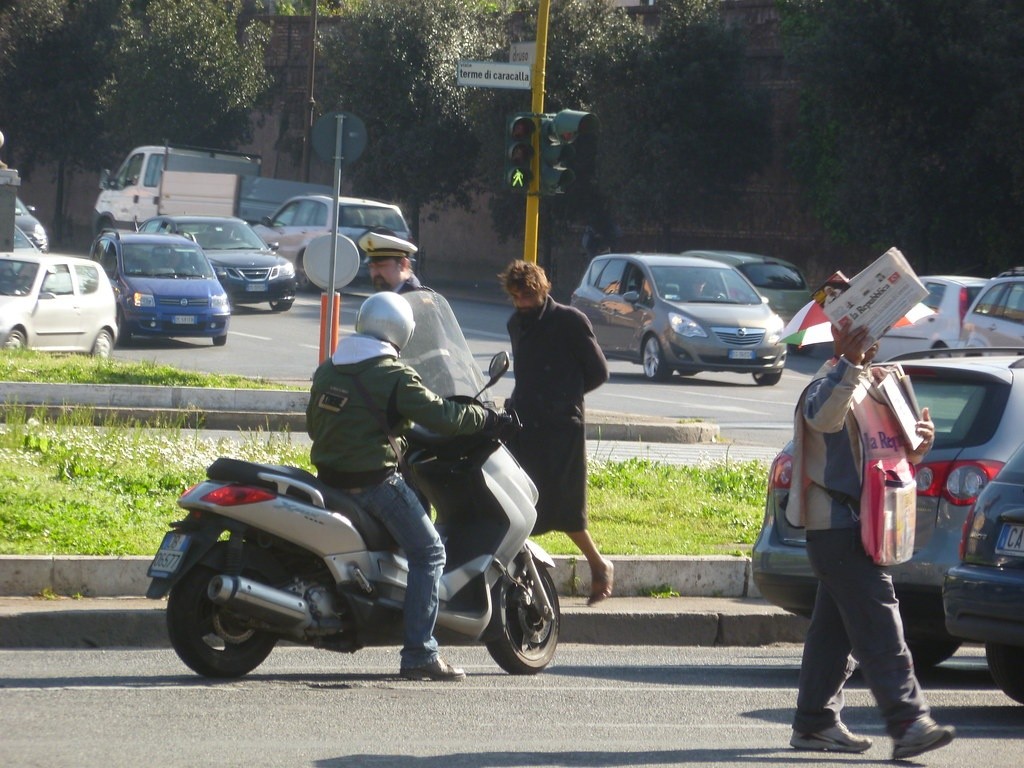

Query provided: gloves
[482,405,508,432]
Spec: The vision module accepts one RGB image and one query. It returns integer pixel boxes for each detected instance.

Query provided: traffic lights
[540,109,601,205]
[504,112,536,196]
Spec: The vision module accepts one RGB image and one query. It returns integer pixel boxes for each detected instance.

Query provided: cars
[682,250,814,356]
[134,214,296,311]
[0,251,118,356]
[12,195,49,251]
[867,275,990,364]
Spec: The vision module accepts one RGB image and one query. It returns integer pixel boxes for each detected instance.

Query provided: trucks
[92,146,336,238]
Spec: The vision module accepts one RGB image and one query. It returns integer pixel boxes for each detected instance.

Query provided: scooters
[146,350,561,680]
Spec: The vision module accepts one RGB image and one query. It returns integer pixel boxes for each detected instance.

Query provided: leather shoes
[400,654,466,682]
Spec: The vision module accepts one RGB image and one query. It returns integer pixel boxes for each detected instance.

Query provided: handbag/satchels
[858,460,917,566]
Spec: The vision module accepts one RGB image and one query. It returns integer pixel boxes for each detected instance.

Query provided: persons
[789,324,957,764]
[357,232,455,522]
[306,292,508,681]
[503,260,614,608]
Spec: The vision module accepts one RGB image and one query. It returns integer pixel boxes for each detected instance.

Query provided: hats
[358,226,418,264]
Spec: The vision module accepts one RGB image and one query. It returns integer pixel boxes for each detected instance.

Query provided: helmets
[355,291,416,352]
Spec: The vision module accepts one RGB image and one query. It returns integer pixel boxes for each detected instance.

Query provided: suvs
[753,347,1024,707]
[958,266,1024,348]
[90,228,229,345]
[252,193,412,294]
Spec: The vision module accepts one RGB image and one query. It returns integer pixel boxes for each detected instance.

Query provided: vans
[570,253,787,386]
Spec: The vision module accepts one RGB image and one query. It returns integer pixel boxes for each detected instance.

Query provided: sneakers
[892,715,956,759]
[789,718,872,752]
